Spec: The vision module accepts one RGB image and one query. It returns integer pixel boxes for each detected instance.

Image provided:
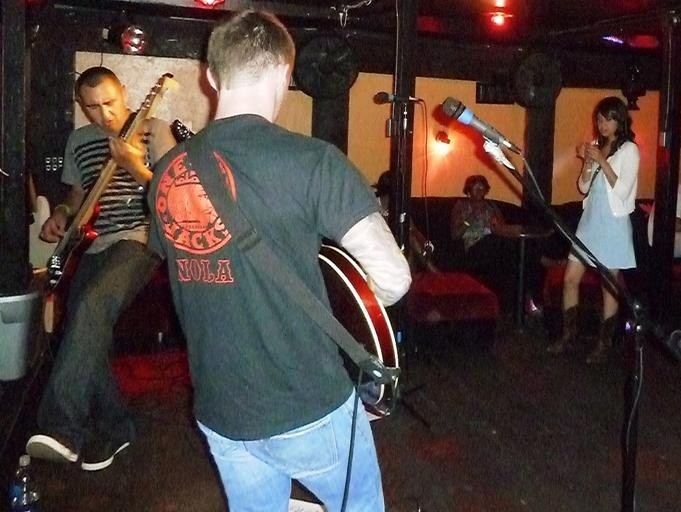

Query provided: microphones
[442,97,523,157]
[372,92,421,105]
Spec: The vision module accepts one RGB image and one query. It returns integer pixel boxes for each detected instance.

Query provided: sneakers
[80,427,129,471]
[26,434,79,464]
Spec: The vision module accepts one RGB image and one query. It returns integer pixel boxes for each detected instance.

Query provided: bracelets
[464,221,472,227]
[55,204,71,215]
[585,168,592,172]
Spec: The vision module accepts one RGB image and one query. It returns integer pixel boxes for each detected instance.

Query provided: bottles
[583,131,604,174]
[8,454,44,512]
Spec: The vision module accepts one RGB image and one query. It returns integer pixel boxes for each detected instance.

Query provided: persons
[647,180,681,258]
[147,8,415,511]
[546,96,643,375]
[25,168,39,262]
[368,170,441,272]
[23,65,180,472]
[449,174,545,318]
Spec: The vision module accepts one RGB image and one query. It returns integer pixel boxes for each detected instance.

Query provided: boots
[547,304,578,353]
[585,313,619,368]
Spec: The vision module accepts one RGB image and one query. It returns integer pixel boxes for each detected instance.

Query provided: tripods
[375,114,436,429]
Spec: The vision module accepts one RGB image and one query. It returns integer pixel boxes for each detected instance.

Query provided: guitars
[46,73,178,294]
[170,119,401,415]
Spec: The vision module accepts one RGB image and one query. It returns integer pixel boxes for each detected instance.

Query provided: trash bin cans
[0,290,39,381]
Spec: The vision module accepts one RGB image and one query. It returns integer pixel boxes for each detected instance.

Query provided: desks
[494,223,555,335]
